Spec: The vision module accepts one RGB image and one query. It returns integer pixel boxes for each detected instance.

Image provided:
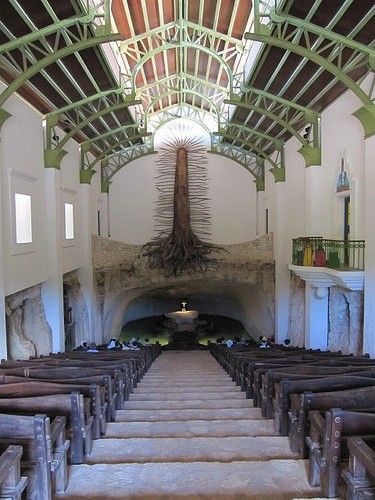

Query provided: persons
[216,335,291,350]
[78,336,150,354]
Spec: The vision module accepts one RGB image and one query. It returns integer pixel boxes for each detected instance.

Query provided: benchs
[208,337,375,500]
[0,338,162,499]
